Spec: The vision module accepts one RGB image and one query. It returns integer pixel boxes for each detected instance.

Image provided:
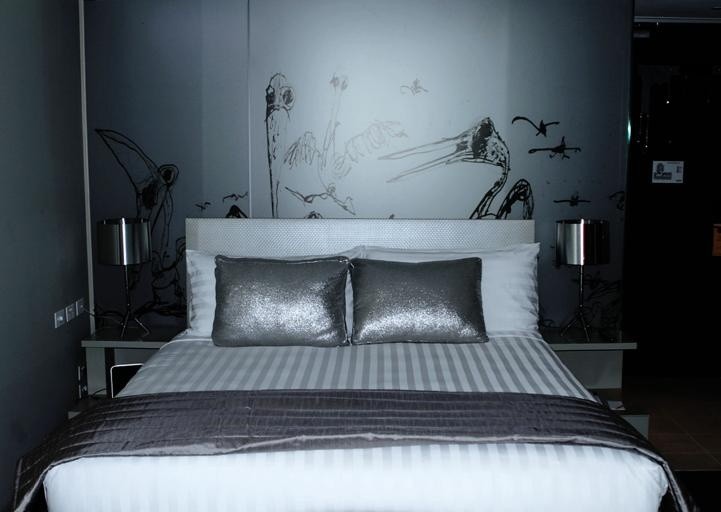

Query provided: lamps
[97,218,158,339]
[556,219,611,342]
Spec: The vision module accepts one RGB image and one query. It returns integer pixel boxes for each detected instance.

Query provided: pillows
[211,254,350,348]
[349,257,489,345]
[364,243,540,335]
[186,246,362,343]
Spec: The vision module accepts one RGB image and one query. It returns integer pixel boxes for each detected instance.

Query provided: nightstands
[81,324,182,396]
[543,327,639,391]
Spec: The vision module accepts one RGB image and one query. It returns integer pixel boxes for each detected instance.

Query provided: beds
[43,217,673,511]
